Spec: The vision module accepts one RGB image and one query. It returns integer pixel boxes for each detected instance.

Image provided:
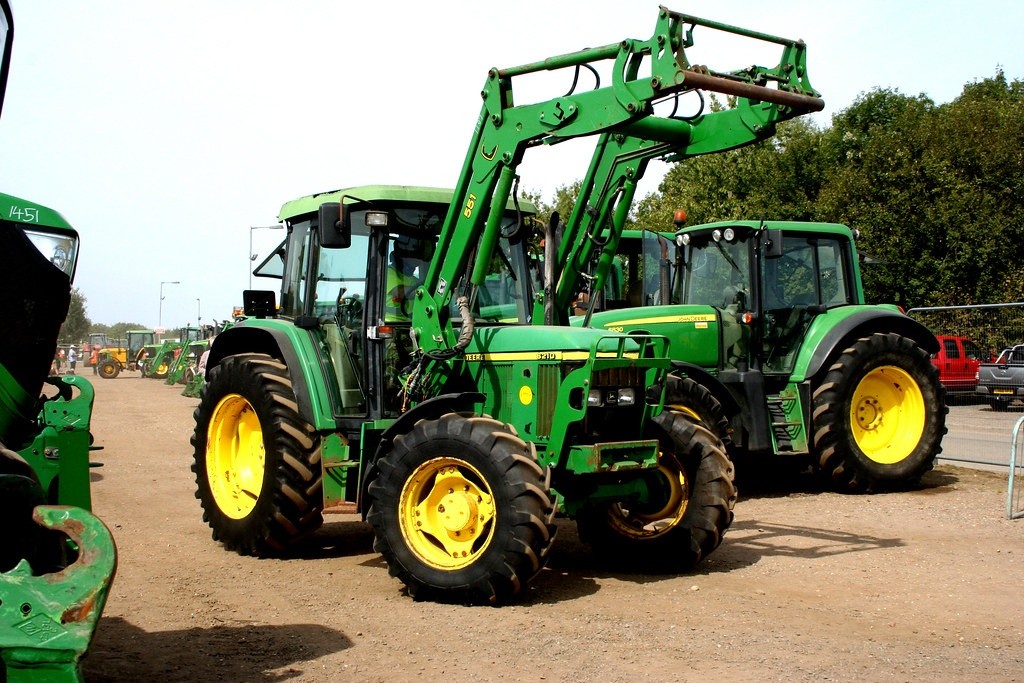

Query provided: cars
[930,336,1006,404]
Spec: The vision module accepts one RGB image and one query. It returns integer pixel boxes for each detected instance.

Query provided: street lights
[158,281,181,328]
[249,225,285,290]
[196,297,200,326]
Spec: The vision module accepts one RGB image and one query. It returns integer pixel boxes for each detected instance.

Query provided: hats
[70,345,76,348]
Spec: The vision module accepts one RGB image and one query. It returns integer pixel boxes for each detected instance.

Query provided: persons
[383,238,422,322]
[89,347,99,377]
[55,344,79,374]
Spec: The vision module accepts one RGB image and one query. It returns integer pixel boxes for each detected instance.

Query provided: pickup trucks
[974,344,1024,411]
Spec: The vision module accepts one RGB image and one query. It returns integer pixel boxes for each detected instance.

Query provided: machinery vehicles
[83,203,951,495]
[0,0,121,683]
[189,7,826,608]
